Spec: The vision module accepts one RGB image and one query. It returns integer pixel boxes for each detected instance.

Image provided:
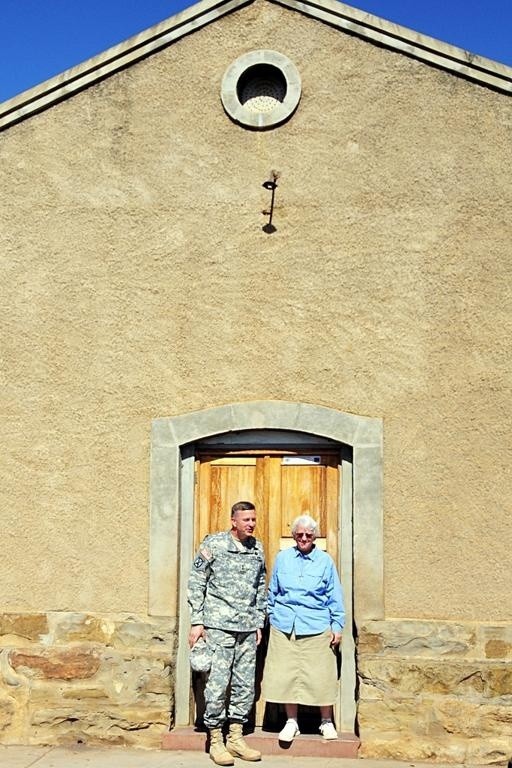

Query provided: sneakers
[278,719,301,742]
[320,720,338,740]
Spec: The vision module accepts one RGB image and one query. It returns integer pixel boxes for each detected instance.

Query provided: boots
[208,728,234,765]
[226,723,261,761]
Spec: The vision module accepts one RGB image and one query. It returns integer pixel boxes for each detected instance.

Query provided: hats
[190,638,212,673]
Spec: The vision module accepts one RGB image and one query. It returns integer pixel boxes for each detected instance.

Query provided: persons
[260,511,346,743]
[185,501,271,767]
